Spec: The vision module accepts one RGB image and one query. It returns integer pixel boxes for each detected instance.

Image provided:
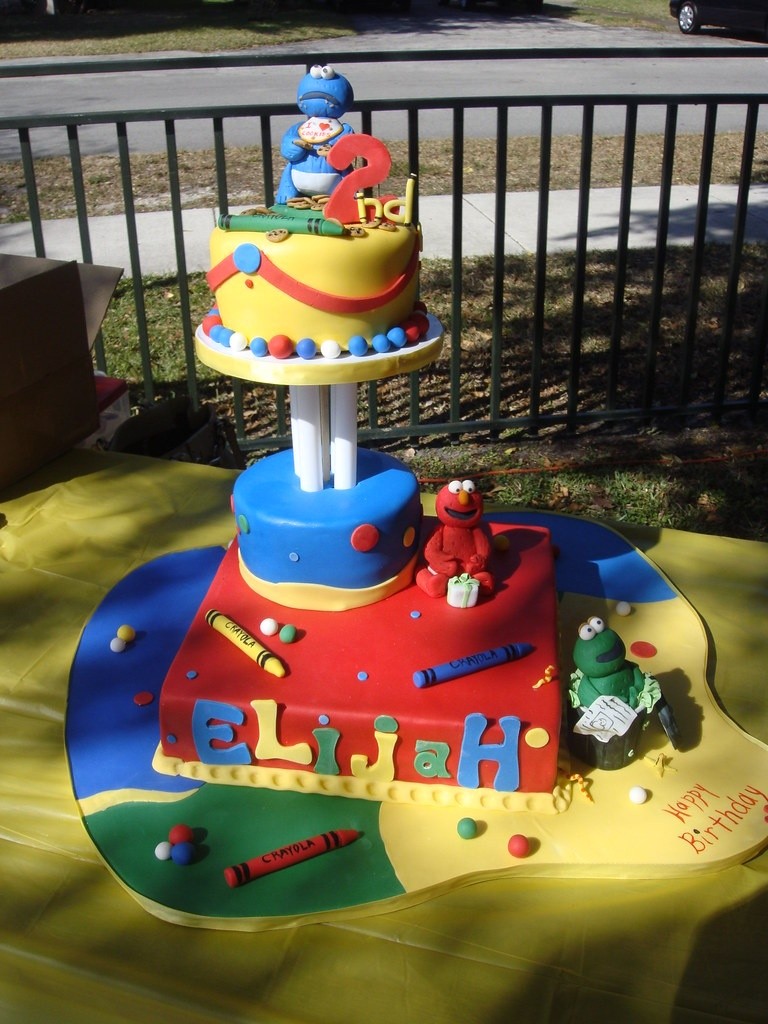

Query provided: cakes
[199,63,430,360]
[151,440,576,818]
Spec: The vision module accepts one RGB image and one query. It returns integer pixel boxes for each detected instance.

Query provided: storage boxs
[0,253,131,494]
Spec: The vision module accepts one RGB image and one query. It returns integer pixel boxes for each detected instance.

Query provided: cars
[668,0,768,45]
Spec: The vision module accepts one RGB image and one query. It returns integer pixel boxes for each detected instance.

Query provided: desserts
[566,616,682,771]
